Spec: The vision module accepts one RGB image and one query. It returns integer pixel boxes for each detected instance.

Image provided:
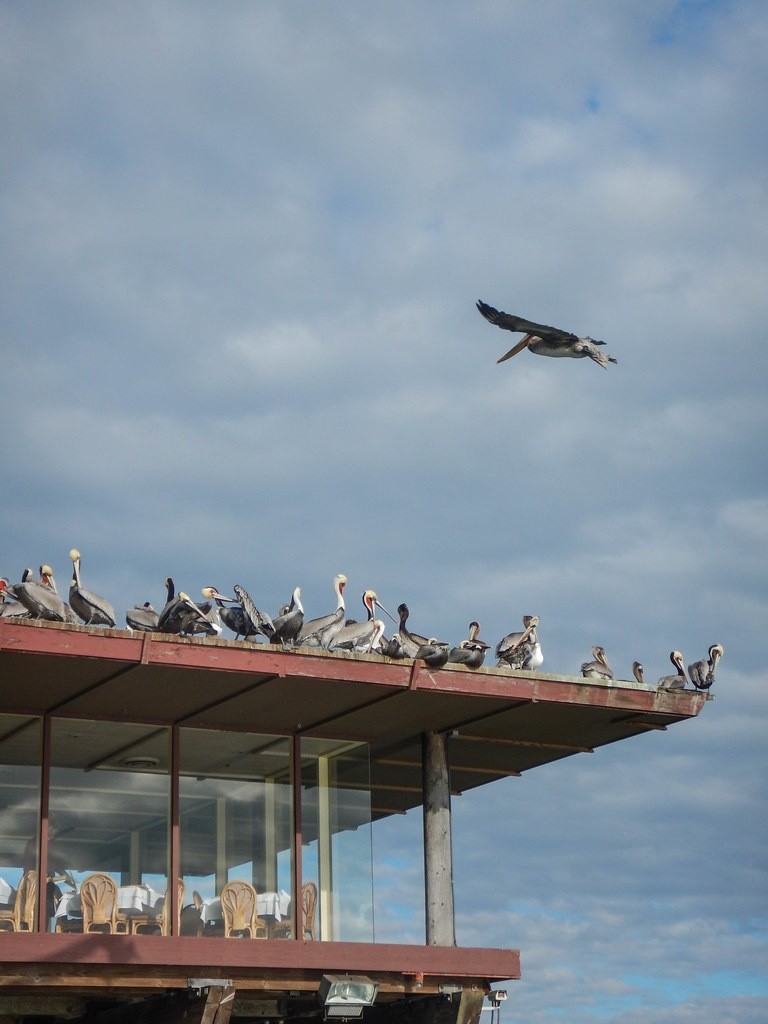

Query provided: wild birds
[658,650,689,689]
[617,661,644,683]
[688,643,724,693]
[473,300,617,370]
[0,549,616,681]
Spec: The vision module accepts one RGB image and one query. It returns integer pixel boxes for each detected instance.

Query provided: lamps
[317,973,380,1019]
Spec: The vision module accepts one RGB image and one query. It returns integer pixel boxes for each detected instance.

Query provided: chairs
[0,870,318,940]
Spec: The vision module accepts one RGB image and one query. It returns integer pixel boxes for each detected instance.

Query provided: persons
[23,816,76,932]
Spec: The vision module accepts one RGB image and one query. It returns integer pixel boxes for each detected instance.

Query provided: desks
[54,884,165,934]
[201,893,291,938]
